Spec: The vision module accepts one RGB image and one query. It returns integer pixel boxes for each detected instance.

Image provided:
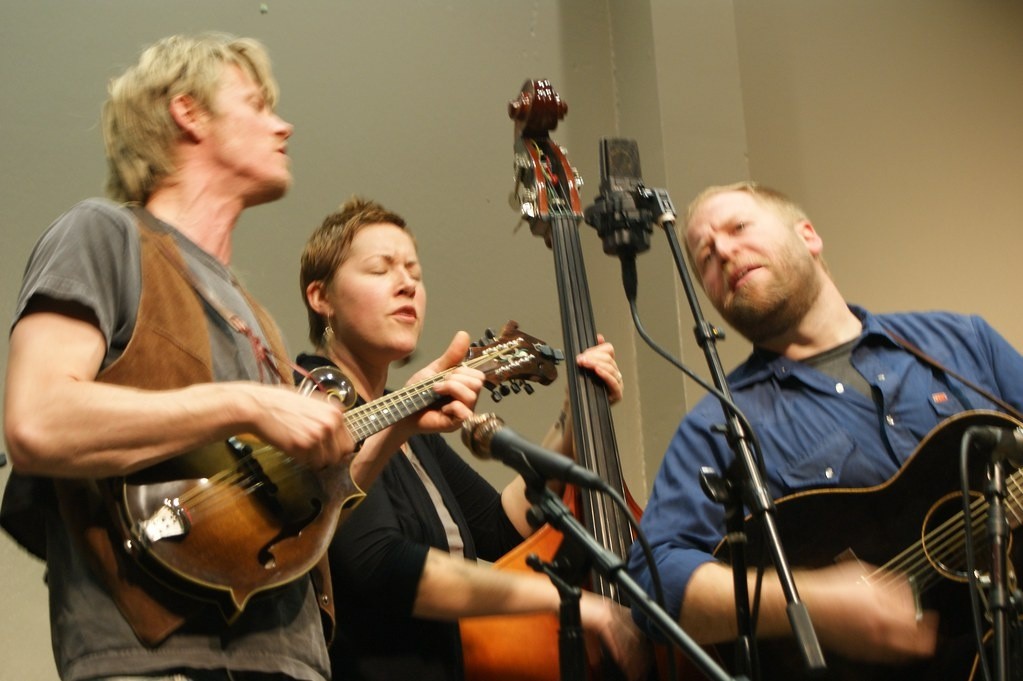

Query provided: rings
[616,373,622,383]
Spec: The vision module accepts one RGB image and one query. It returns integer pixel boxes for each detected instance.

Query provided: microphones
[600,134,644,302]
[461,412,608,493]
[971,424,1023,468]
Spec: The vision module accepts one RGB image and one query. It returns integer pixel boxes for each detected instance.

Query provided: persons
[293,197,654,681]
[0,30,485,681]
[626,182,1022,680]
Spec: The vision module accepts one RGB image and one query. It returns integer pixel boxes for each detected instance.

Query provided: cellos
[445,72,730,681]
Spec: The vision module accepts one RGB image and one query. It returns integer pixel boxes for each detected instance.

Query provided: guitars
[110,318,568,629]
[708,406,1022,681]
